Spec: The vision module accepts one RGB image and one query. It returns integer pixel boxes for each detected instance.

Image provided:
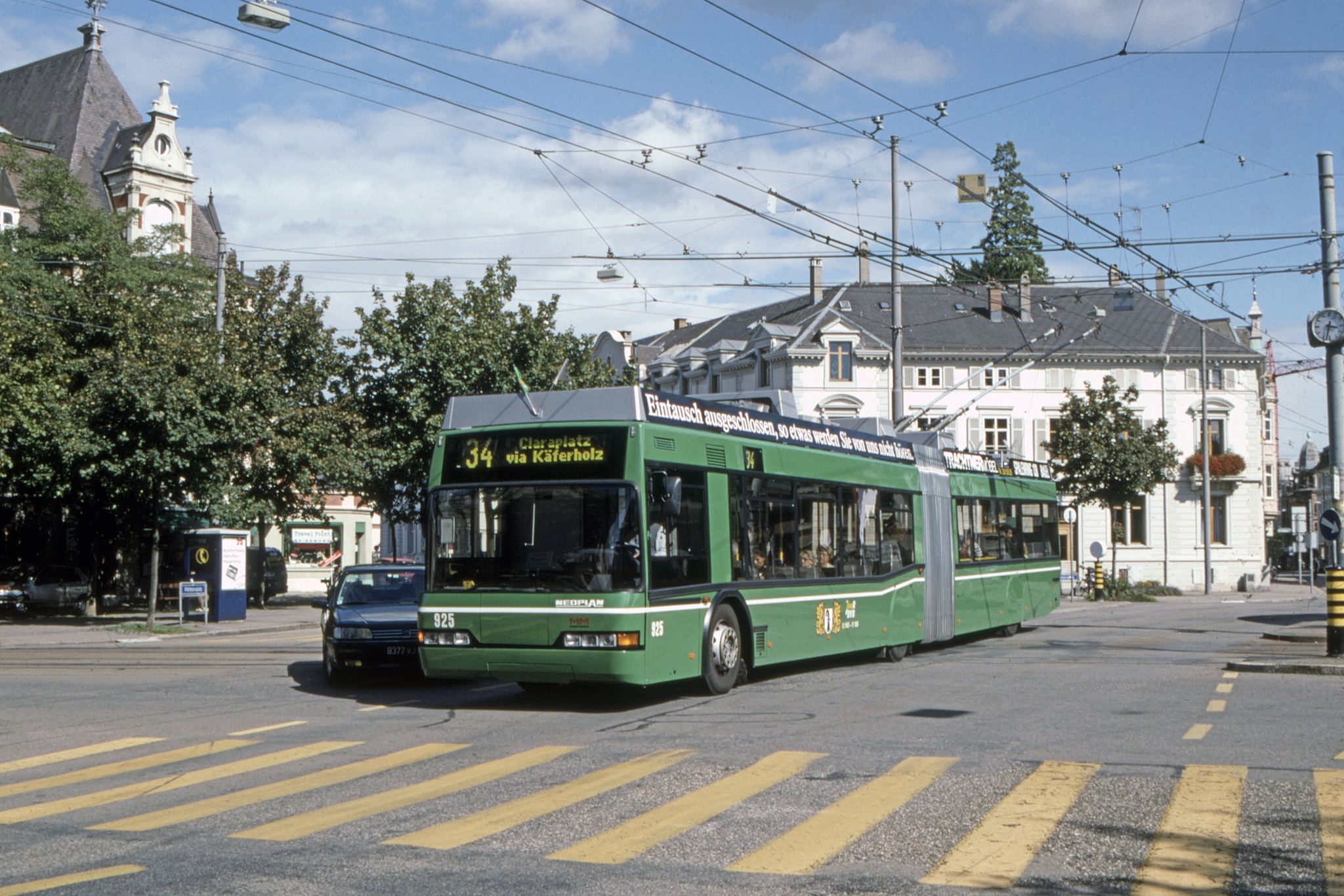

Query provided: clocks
[1310,307,1344,346]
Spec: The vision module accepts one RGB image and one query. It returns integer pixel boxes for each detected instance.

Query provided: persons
[732,512,1028,580]
[619,503,667,556]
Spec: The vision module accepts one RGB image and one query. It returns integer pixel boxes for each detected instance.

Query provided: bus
[376,558,417,583]
[417,386,1062,696]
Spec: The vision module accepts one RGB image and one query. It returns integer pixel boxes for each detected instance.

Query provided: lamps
[596,267,624,282]
[235,3,292,30]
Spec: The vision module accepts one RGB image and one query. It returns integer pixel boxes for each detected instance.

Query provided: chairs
[593,532,1056,580]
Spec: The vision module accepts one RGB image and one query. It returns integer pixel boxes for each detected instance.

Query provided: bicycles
[1077,565,1113,596]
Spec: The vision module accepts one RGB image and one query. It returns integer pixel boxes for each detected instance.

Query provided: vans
[150,547,288,608]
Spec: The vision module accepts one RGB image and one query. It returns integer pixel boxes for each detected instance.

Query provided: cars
[0,565,92,617]
[311,564,427,686]
